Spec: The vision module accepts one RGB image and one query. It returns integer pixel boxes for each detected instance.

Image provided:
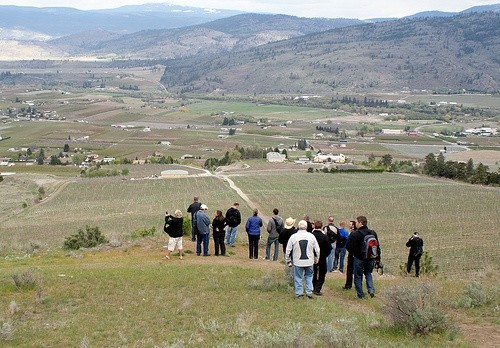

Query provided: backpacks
[357,230,380,261]
[326,226,337,243]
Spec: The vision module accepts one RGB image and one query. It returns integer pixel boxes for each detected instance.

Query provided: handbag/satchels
[272,217,282,233]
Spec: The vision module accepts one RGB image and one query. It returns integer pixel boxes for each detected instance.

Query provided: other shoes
[203,254,211,256]
[338,268,343,273]
[314,292,323,296]
[342,287,349,289]
[332,267,338,271]
[307,296,314,299]
[178,256,182,260]
[197,252,200,256]
[166,255,170,260]
[369,293,374,298]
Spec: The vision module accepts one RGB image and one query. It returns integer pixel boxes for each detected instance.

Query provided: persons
[286,219,320,298]
[405,232,423,278]
[165,209,184,259]
[312,221,332,295]
[245,208,263,260]
[212,210,230,256]
[346,216,381,299]
[263,208,284,261]
[194,204,211,256]
[187,196,202,242]
[225,202,241,247]
[278,215,349,274]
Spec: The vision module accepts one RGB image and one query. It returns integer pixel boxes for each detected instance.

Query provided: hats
[283,217,297,229]
[298,220,308,230]
[200,204,209,210]
[413,232,420,235]
[174,210,183,217]
